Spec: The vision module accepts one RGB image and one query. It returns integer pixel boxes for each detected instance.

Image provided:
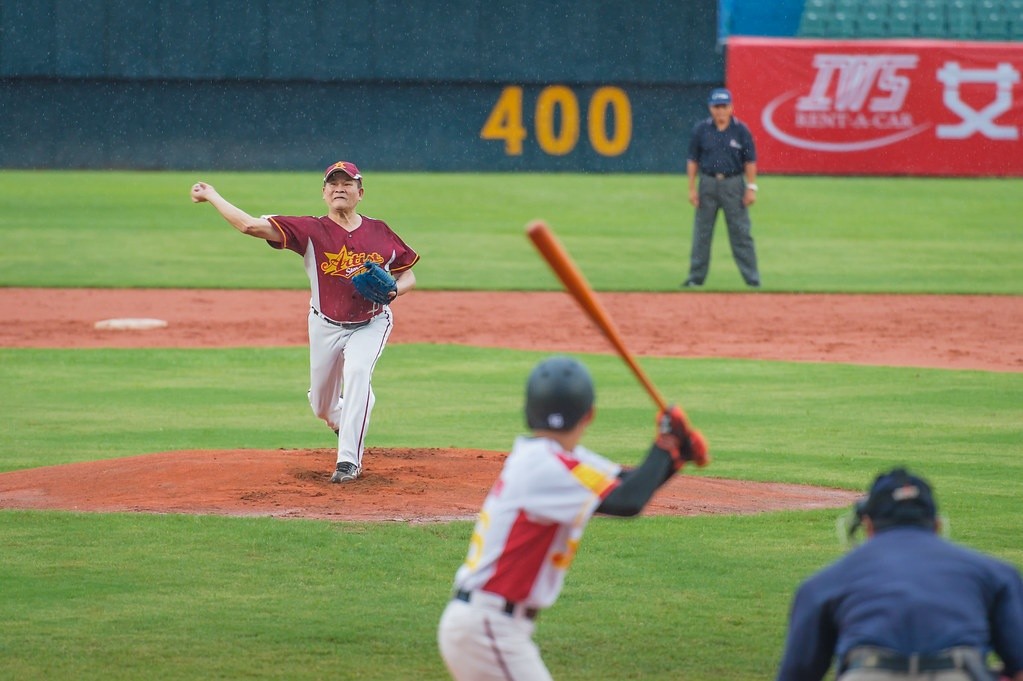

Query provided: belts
[703,171,741,179]
[314,309,371,330]
[841,653,955,670]
[456,592,537,618]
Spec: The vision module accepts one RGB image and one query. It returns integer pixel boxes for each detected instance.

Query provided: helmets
[525,360,594,429]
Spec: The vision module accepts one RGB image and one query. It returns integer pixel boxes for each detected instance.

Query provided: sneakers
[331,462,362,483]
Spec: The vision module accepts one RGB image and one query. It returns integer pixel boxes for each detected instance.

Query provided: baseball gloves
[352,260,397,307]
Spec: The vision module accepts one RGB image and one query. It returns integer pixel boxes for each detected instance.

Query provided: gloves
[656,405,709,471]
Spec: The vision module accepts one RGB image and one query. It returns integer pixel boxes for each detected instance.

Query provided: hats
[855,470,936,517]
[324,160,362,185]
[708,88,731,105]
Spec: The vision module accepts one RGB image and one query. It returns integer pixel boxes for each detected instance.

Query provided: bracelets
[746,184,757,191]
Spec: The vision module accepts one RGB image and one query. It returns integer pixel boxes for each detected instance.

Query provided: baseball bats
[526,221,707,469]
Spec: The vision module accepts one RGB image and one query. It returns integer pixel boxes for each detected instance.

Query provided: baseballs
[191,184,203,192]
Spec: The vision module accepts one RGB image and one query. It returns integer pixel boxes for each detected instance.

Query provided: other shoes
[683,280,702,287]
[747,280,759,286]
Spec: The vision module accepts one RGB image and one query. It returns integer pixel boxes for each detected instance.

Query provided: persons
[436,356,707,681]
[190,161,421,483]
[679,88,761,288]
[777,462,1023,681]
